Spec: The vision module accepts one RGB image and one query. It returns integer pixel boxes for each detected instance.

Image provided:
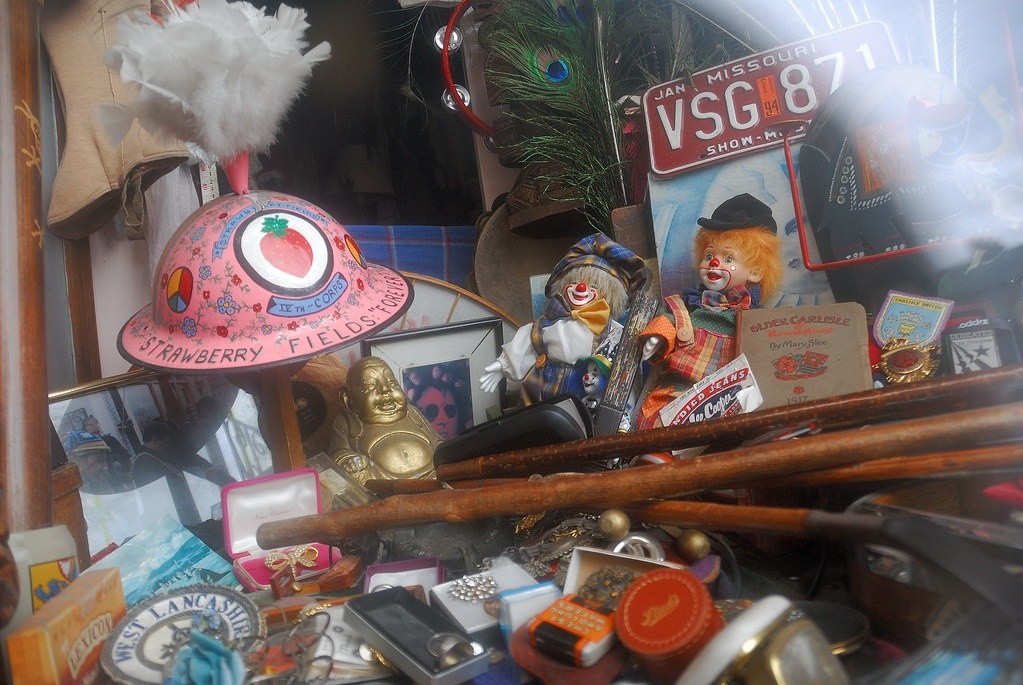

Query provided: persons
[400,365,465,443]
[478,233,652,407]
[62,399,265,540]
[634,195,778,460]
[329,355,457,494]
[558,353,632,435]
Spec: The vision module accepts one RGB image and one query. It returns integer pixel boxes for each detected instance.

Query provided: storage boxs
[346,547,692,685]
[8,568,126,685]
[222,469,344,592]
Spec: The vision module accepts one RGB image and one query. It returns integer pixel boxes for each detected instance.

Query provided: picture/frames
[48,356,275,570]
[362,316,508,442]
